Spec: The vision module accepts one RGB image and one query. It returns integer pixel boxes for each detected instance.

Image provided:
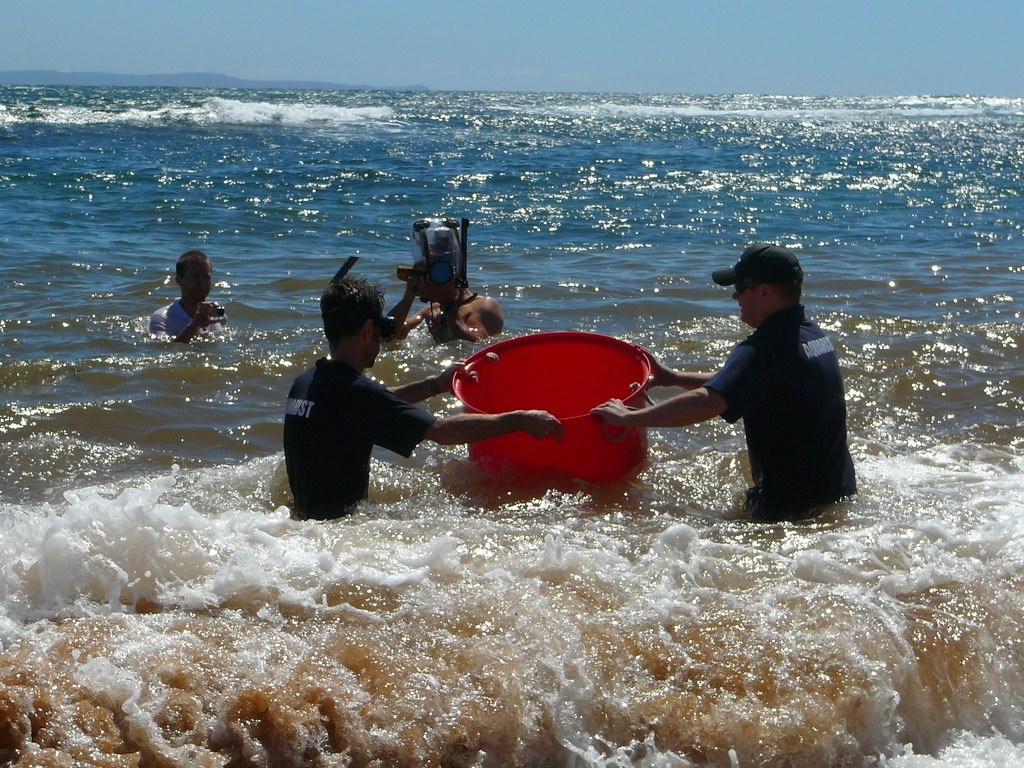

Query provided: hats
[711,243,804,287]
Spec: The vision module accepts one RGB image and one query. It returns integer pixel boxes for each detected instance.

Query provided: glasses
[733,280,751,294]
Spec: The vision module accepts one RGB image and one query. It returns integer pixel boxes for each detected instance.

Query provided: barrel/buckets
[453,332,653,487]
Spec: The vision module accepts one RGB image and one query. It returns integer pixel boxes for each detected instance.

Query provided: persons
[591,243,860,518]
[281,278,566,517]
[147,250,229,343]
[385,286,504,342]
